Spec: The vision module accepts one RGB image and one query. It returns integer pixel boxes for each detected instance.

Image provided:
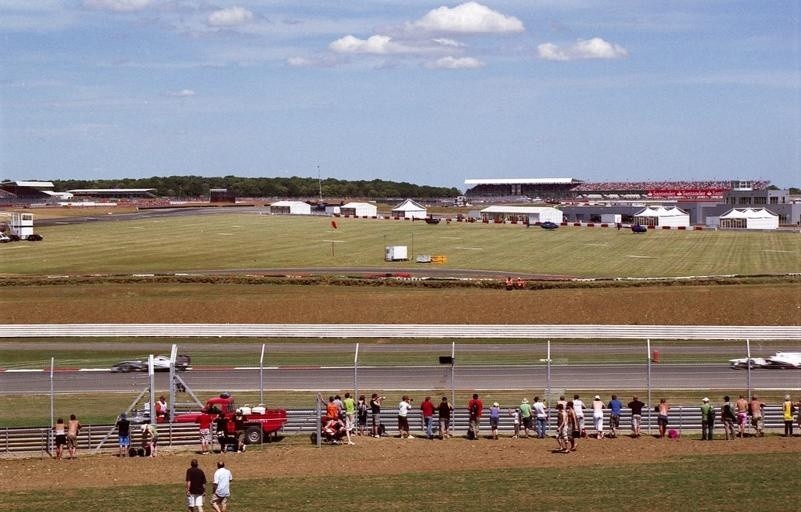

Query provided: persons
[489,401,500,440]
[156,396,170,420]
[232,409,248,453]
[509,395,548,439]
[214,413,229,453]
[320,393,385,446]
[67,414,81,459]
[195,409,212,454]
[186,459,207,512]
[420,396,455,440]
[700,397,714,440]
[144,399,150,417]
[655,398,670,439]
[116,413,130,457]
[721,394,766,440]
[556,394,586,453]
[398,394,415,439]
[210,461,232,512]
[591,394,605,440]
[469,394,483,440]
[783,394,795,437]
[140,424,159,457]
[607,394,622,438]
[52,418,67,460]
[628,394,645,438]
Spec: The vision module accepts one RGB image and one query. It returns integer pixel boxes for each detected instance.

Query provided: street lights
[316,164,322,202]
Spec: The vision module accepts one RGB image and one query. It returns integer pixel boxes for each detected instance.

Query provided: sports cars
[726,349,800,371]
[111,350,192,373]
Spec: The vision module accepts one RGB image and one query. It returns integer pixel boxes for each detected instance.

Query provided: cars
[631,225,647,233]
[425,216,441,224]
[541,221,558,229]
[0,232,44,244]
[456,213,465,221]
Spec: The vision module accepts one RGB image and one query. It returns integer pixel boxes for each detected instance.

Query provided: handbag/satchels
[791,406,797,413]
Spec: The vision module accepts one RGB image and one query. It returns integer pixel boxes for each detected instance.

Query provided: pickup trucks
[168,396,285,446]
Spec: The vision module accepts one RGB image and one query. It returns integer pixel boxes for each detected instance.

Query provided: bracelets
[188,490,190,491]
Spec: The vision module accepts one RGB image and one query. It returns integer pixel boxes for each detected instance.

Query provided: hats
[595,395,601,399]
[521,398,530,404]
[701,396,710,402]
[783,395,792,400]
[119,413,128,420]
[492,402,499,407]
